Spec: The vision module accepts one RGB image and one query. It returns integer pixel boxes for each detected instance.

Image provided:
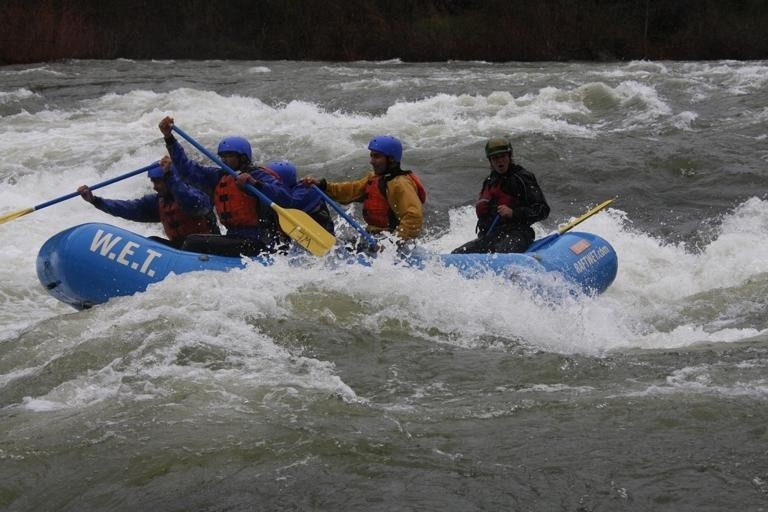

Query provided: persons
[297,135,427,259]
[450,135,551,254]
[77,116,337,258]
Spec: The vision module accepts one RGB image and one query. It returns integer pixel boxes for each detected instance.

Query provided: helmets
[486,136,512,161]
[218,136,251,162]
[148,159,172,178]
[265,161,296,186]
[368,135,402,163]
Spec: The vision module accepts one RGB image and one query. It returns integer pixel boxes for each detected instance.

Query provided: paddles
[0,161,161,224]
[169,123,337,258]
[525,200,613,253]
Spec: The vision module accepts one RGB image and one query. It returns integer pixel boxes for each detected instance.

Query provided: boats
[35,223,618,316]
